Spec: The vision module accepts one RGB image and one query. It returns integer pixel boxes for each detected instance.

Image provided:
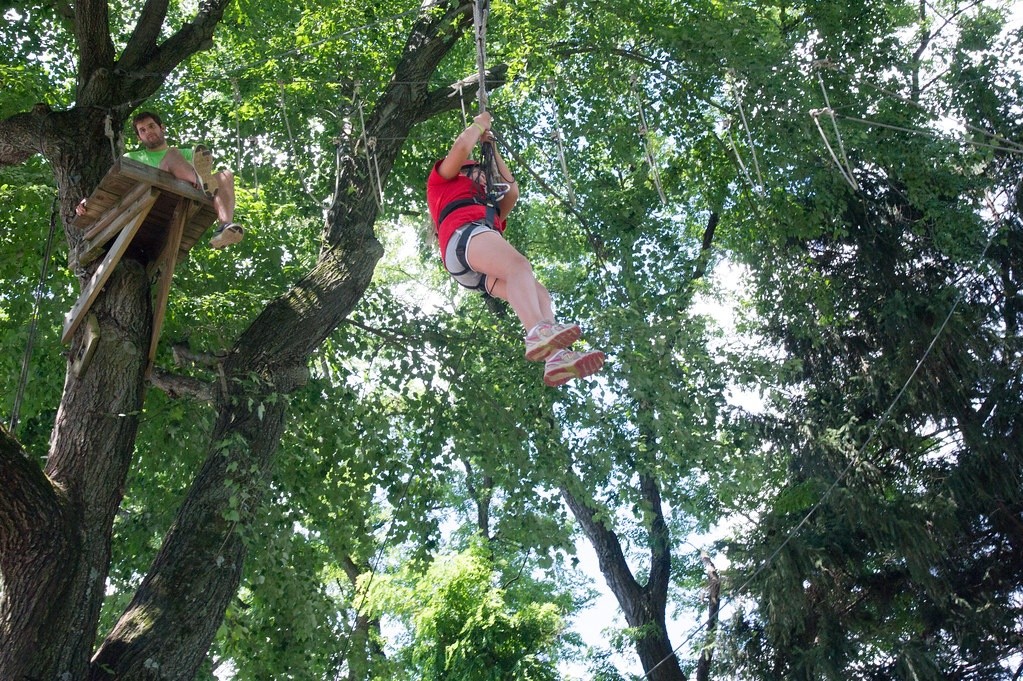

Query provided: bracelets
[472,122,483,135]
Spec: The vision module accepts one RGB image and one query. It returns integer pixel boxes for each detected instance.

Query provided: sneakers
[544,349,605,388]
[207,222,244,249]
[524,321,581,363]
[193,143,219,198]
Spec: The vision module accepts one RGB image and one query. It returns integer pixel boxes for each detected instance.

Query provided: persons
[76,112,244,248]
[427,111,605,385]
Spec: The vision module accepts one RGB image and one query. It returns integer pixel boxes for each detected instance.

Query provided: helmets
[461,158,483,170]
